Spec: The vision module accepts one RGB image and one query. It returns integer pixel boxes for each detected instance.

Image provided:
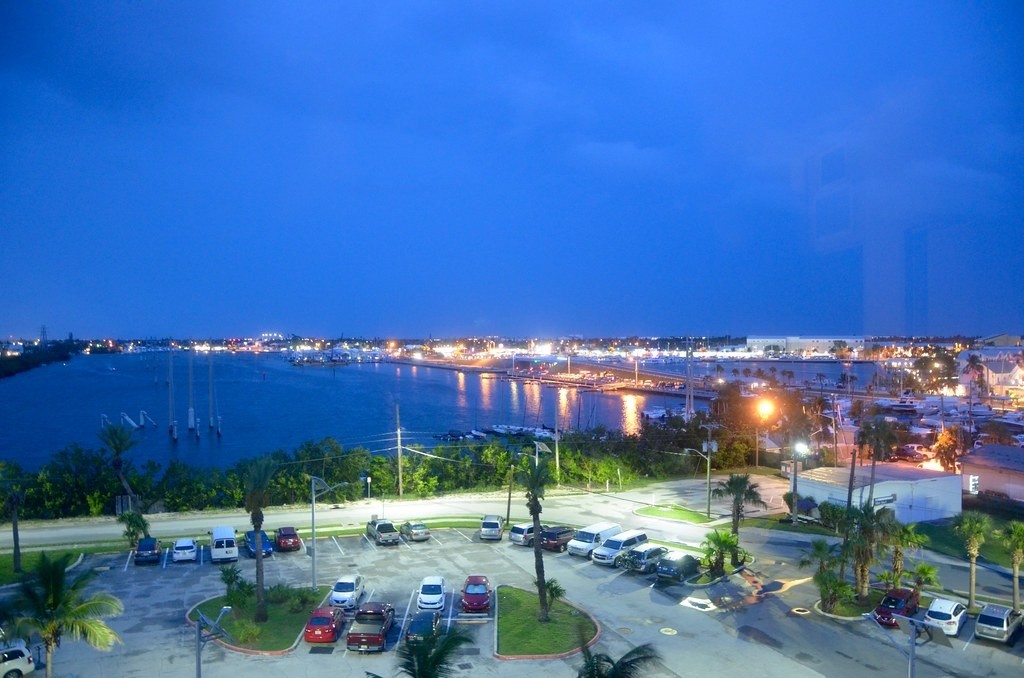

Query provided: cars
[623,378,686,389]
[171,538,198,562]
[399,521,431,540]
[461,575,494,614]
[873,588,920,629]
[926,598,968,638]
[417,576,448,616]
[136,537,162,566]
[872,396,1024,463]
[330,574,366,609]
[274,527,302,552]
[243,529,272,560]
[974,603,1023,648]
[304,607,345,644]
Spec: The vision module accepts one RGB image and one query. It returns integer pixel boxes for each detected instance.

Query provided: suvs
[480,515,505,541]
[538,526,577,553]
[509,522,551,548]
[657,552,702,583]
[592,530,649,568]
[0,639,36,678]
[629,544,674,574]
[567,521,623,561]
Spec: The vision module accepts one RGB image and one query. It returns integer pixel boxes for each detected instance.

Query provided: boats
[433,423,563,444]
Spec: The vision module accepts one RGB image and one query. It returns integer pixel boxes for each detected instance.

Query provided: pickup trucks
[345,601,395,653]
[368,519,400,547]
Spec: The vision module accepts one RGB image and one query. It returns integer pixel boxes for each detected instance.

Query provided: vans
[208,526,240,563]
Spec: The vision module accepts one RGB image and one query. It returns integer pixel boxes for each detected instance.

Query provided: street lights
[310,475,348,596]
[517,451,539,470]
[754,401,765,470]
[367,476,372,501]
[684,447,711,519]
[792,440,805,525]
